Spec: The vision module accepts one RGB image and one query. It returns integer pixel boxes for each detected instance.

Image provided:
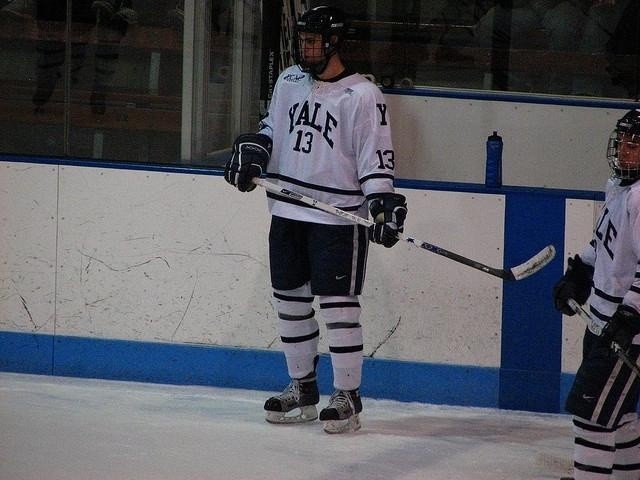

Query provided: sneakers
[262,371,320,413]
[317,387,363,421]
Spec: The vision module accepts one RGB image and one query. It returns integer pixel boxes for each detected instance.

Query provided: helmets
[605,107,639,183]
[291,6,344,66]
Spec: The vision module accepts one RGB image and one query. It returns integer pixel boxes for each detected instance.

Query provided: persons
[348,4,423,89]
[222,2,408,422]
[30,1,135,123]
[551,109,639,480]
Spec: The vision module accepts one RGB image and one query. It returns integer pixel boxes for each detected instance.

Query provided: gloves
[593,302,640,367]
[365,191,409,251]
[222,131,273,195]
[551,252,595,319]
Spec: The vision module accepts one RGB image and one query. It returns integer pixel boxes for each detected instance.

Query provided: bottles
[485,130,504,188]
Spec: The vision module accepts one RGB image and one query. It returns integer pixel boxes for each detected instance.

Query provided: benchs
[341,0,640,101]
[0,0,262,153]
